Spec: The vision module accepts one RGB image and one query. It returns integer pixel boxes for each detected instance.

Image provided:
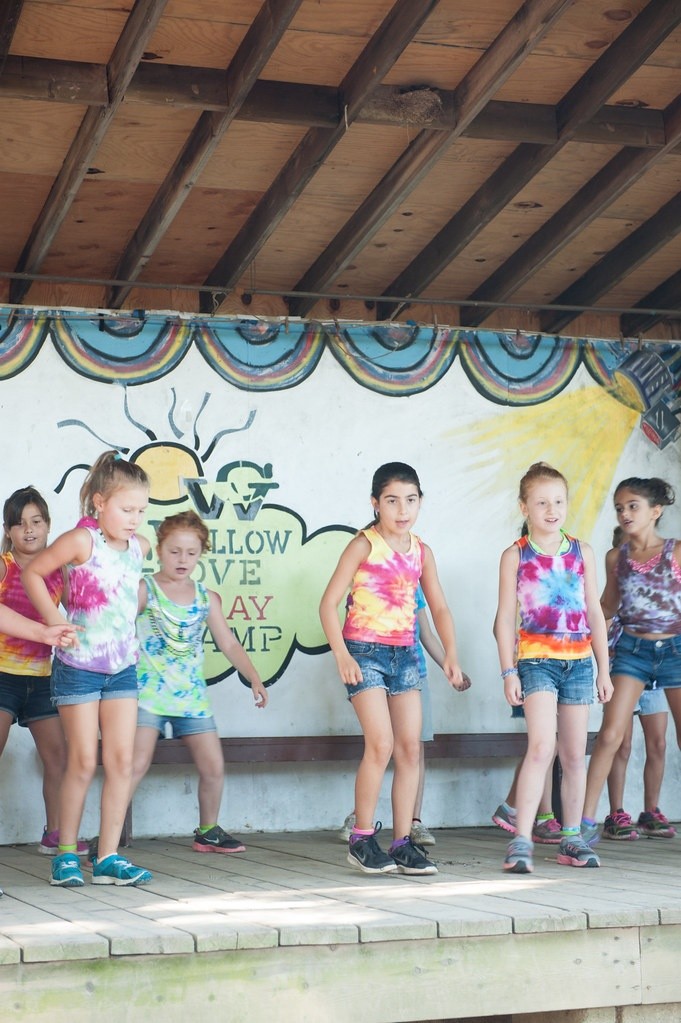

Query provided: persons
[20,450,152,886]
[495,462,681,873]
[319,461,461,874]
[86,509,268,864]
[0,486,110,855]
[339,488,472,844]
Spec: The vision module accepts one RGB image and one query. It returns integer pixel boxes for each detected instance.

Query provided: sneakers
[603,811,639,840]
[581,824,600,845]
[86,836,99,867]
[636,812,674,839]
[48,853,84,886]
[411,824,435,845]
[40,825,89,855]
[492,803,517,833]
[503,837,533,873]
[347,821,397,873]
[556,833,601,867]
[340,814,356,842]
[532,818,562,843]
[387,836,438,875]
[192,825,246,852]
[91,856,153,885]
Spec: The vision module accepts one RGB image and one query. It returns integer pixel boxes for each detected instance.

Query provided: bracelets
[501,668,518,679]
